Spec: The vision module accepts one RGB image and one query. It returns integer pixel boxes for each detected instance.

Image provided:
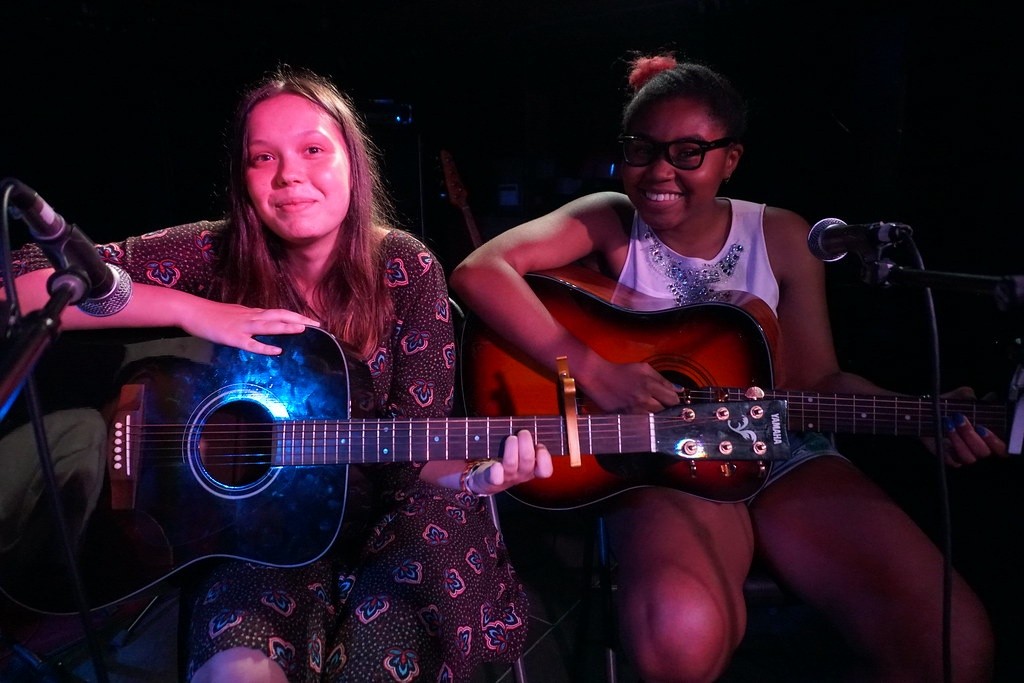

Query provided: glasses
[616,133,738,170]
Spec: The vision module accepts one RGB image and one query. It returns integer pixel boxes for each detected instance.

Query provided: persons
[0,60,531,683]
[449,57,1007,683]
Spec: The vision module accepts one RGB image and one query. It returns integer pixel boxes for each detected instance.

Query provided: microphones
[806,218,911,262]
[0,180,132,315]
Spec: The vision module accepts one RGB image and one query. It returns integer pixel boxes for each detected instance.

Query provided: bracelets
[459,460,496,500]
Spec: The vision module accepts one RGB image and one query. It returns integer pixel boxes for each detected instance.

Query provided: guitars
[1,320,793,641]
[457,263,1024,515]
[432,148,482,251]
